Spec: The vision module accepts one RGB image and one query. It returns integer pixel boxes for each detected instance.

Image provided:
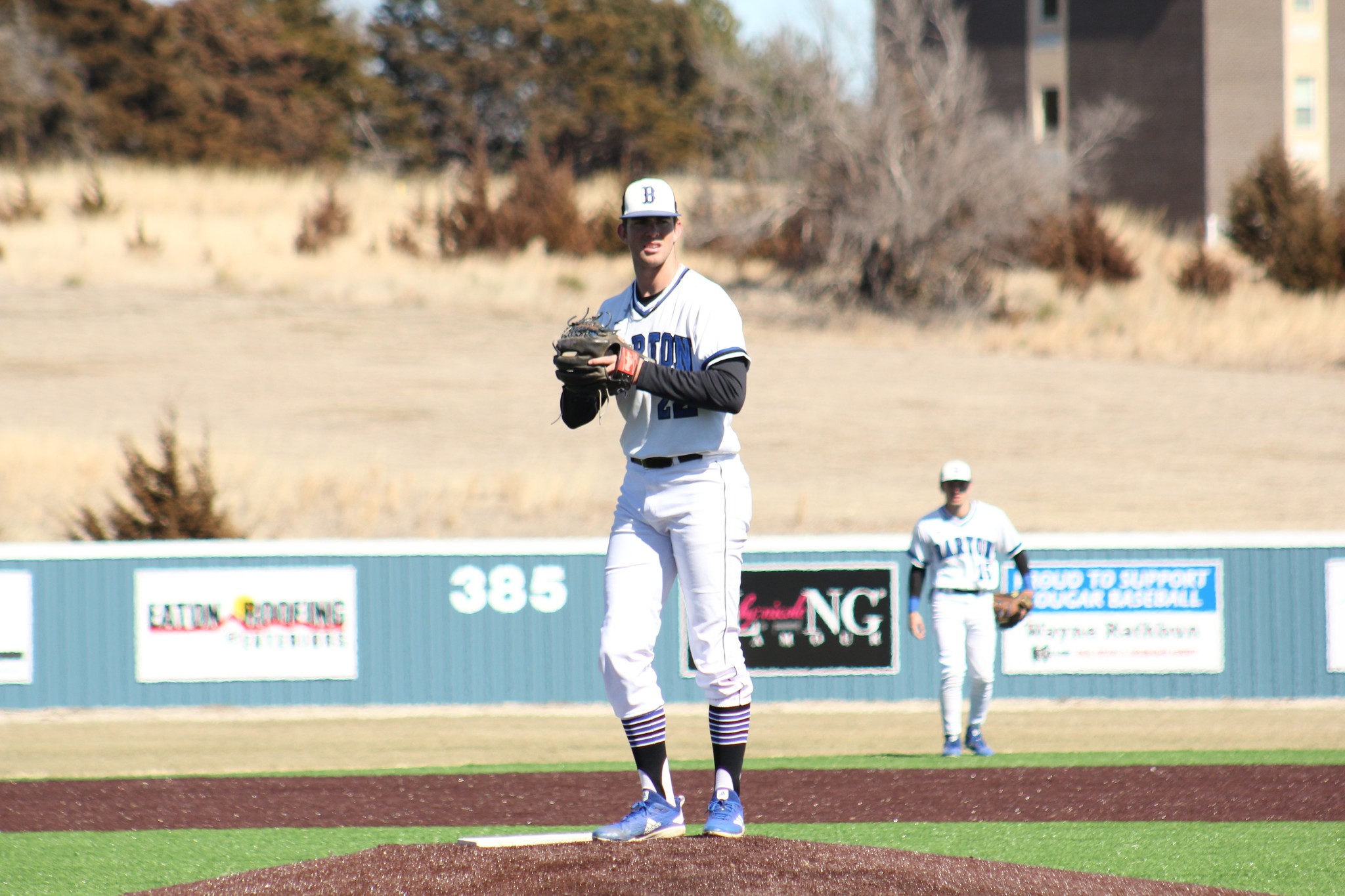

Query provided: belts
[631,453,703,469]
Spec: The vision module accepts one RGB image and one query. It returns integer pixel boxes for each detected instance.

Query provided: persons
[552,177,755,843]
[906,460,1033,758]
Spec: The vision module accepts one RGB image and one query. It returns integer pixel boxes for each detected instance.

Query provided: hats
[940,460,971,482]
[620,178,681,218]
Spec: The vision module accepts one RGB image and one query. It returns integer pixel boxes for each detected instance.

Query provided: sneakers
[592,788,686,840]
[703,787,745,838]
[942,736,961,758]
[964,728,991,757]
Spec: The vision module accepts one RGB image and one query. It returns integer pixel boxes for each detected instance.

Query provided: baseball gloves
[994,592,1034,628]
[550,316,641,394]
